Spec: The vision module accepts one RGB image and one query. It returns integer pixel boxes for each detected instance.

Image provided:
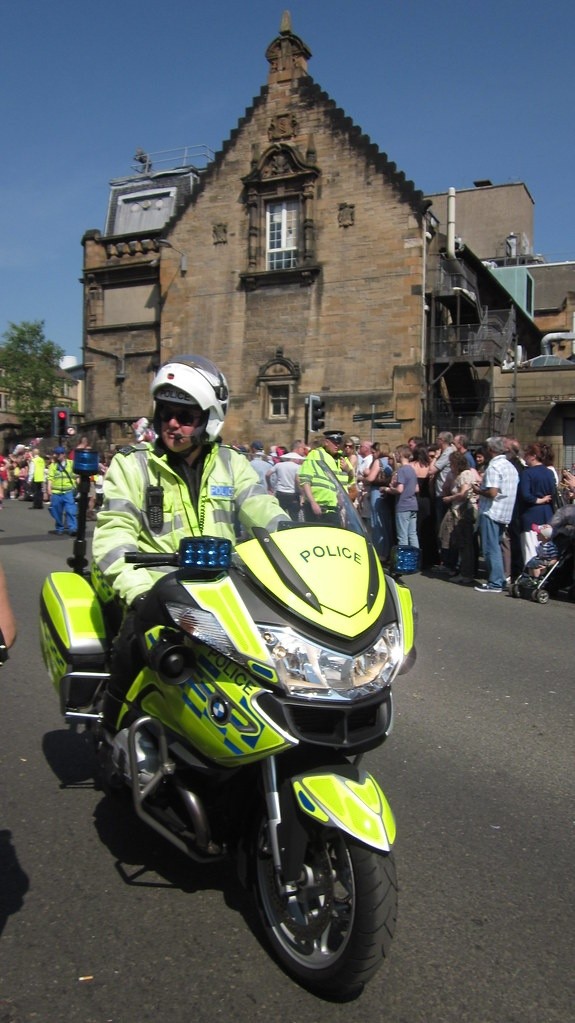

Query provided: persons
[0,564,19,671]
[92,352,295,792]
[0,435,109,537]
[235,432,575,593]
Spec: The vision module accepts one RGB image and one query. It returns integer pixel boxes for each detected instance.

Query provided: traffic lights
[306,394,326,433]
[53,406,69,437]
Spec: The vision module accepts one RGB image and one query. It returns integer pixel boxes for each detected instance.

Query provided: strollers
[508,503,575,604]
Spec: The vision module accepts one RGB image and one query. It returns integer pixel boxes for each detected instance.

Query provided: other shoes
[56,529,64,534]
[432,565,451,573]
[29,505,43,509]
[71,531,78,537]
[448,574,475,583]
[519,577,540,589]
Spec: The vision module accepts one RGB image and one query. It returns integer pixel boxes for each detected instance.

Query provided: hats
[323,431,345,443]
[251,441,264,450]
[532,524,553,540]
[350,436,360,446]
[54,447,65,454]
[280,452,306,460]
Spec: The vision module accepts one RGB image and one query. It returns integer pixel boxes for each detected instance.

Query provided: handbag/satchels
[370,459,385,485]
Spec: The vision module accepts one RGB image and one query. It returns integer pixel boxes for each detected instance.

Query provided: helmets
[153,354,230,444]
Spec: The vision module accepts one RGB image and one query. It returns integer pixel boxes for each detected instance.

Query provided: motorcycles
[34,450,420,1003]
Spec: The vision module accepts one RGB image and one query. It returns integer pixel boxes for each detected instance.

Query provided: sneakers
[475,582,503,593]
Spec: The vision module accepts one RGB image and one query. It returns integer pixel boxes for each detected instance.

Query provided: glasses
[435,437,442,441]
[429,455,434,459]
[158,405,203,426]
[344,444,353,449]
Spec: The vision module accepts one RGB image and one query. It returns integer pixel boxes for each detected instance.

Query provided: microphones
[174,433,185,441]
